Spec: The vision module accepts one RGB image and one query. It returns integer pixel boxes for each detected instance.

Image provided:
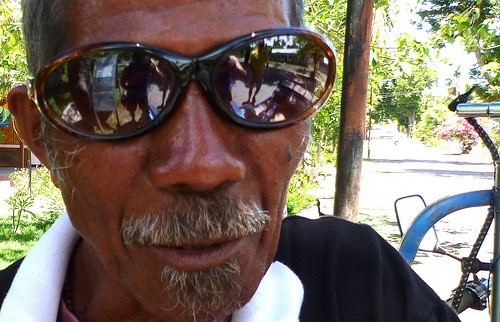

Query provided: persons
[0,0,465,322]
[67,37,274,135]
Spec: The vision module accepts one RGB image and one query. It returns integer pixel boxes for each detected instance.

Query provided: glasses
[25,28,337,141]
